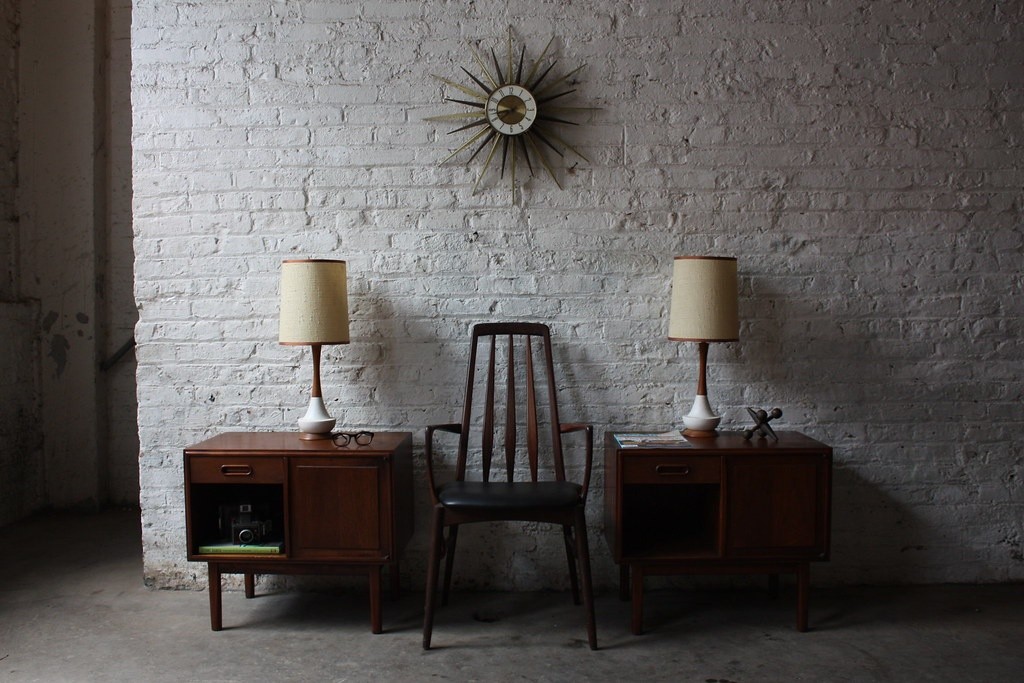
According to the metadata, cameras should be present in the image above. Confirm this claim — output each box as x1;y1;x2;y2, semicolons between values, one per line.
234;517;266;544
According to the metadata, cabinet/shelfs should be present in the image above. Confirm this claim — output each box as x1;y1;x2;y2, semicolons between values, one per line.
184;432;412;634
602;430;833;636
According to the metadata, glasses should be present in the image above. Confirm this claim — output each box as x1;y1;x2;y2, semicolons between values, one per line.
332;431;374;447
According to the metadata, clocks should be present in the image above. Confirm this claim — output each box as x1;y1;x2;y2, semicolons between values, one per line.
485;83;538;137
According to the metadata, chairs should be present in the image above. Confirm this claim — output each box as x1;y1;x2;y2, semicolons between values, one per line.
422;323;598;651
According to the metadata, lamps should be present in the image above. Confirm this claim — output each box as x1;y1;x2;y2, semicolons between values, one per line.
667;256;741;439
278;259;350;441
